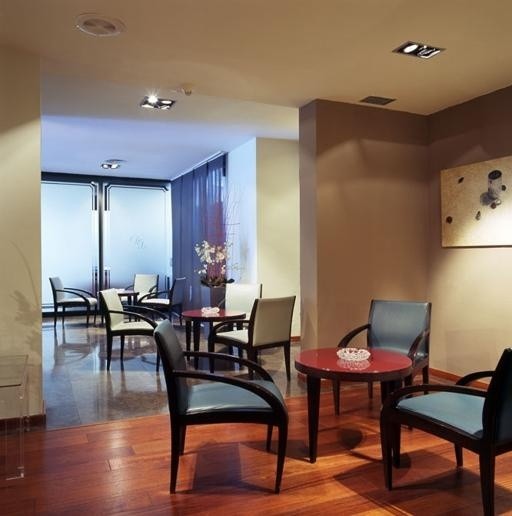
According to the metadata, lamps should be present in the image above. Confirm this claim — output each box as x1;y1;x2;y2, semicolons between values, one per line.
98;160;127;169
139;96;176;110
391;40;445;59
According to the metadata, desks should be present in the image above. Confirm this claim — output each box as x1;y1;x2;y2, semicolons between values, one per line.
0;354;31;482
294;347;414;469
182;309;247;375
96;290;139;326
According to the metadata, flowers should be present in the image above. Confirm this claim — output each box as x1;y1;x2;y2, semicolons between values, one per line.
191;239;240;289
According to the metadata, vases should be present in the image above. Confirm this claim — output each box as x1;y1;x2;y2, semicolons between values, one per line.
200;284;226;314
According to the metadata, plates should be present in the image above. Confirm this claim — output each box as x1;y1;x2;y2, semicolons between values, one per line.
337;348;371;361
201;313;220;317
337;359;370;371
201;307;220;313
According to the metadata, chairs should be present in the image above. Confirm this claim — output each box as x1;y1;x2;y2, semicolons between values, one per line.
138;277;186;326
209;283;263;354
125;273;159;322
49;276;98;328
378;347;512;516
207;295;296;382
99;288;170;374
153;317;290;495
334;299;432;430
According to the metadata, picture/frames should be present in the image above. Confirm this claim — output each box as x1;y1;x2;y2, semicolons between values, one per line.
440;155;512;248
